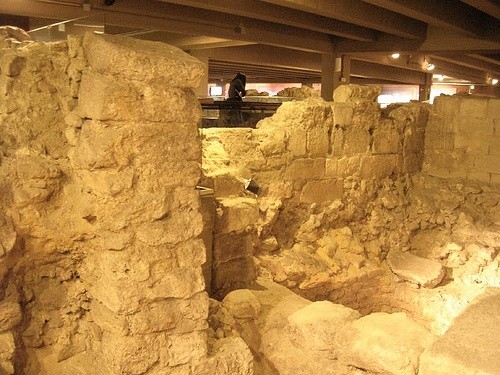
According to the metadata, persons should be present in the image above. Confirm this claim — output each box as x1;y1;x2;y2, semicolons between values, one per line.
229;73;246;109
